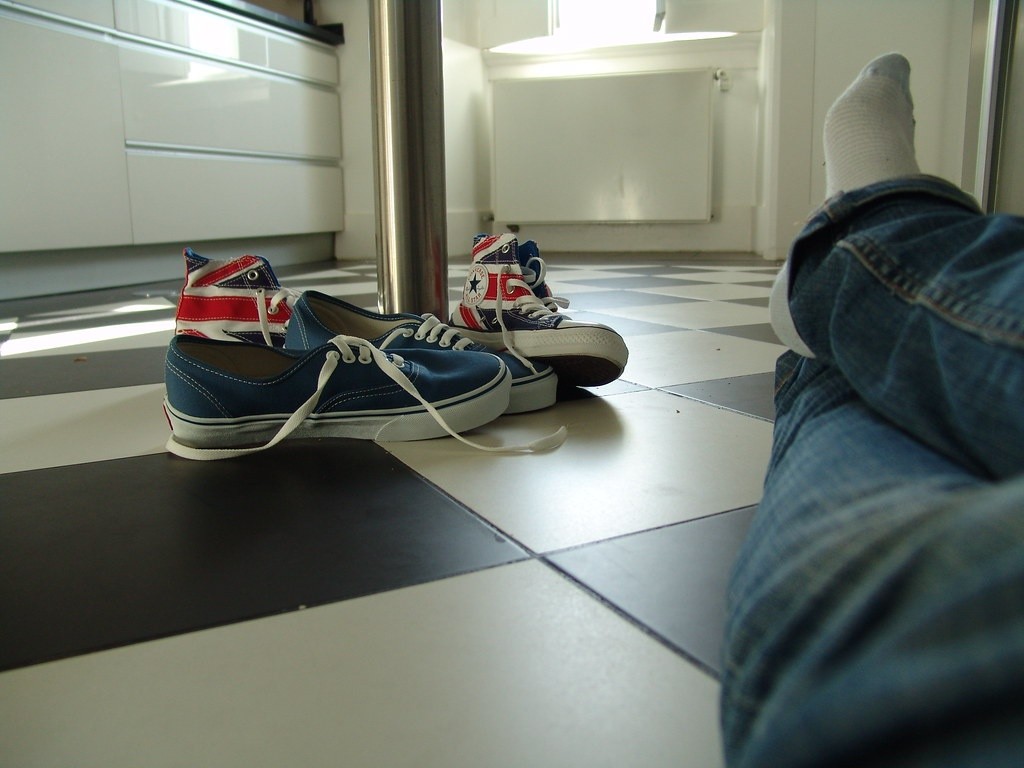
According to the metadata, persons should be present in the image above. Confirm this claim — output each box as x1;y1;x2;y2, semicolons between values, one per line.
721;53;1024;768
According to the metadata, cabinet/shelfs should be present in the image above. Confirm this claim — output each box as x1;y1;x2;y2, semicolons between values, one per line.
1;0;345;303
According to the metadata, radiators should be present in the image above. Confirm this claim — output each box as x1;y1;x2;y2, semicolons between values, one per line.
479;64;730;231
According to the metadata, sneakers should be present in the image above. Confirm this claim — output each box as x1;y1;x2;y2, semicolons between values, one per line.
447;233;629;387
282;290;558;414
175;248;302;350
162;334;512;460
